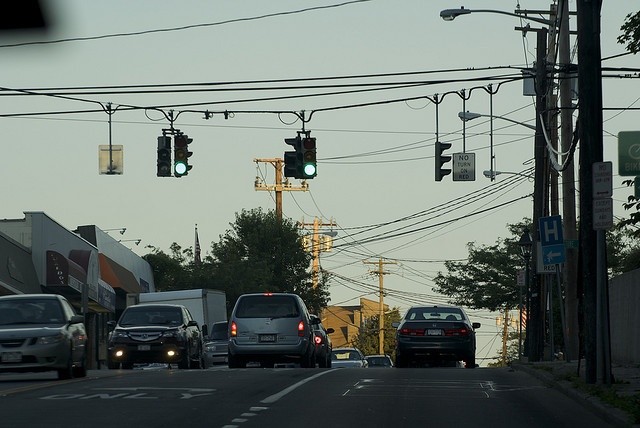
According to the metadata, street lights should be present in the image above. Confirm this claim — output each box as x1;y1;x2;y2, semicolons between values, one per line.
458;112;545;362
484;171;538;359
439;7;569;365
518;228;533;356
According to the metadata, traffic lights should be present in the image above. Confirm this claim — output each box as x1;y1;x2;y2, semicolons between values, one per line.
284;137;301;179
157;136;172;177
301;137;317;179
174;135;187;177
435;142;451;181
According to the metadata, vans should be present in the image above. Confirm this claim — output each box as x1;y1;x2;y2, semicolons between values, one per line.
228;293;321;370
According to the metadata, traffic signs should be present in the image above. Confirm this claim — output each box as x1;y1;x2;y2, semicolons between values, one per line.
539;215;566;264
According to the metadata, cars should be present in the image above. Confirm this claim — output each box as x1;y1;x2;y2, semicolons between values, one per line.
364;354;393;369
309;314;334;369
201;321;229;370
106;303;201;370
392;303;480;368
0;293;88;379
331;348;368;368
